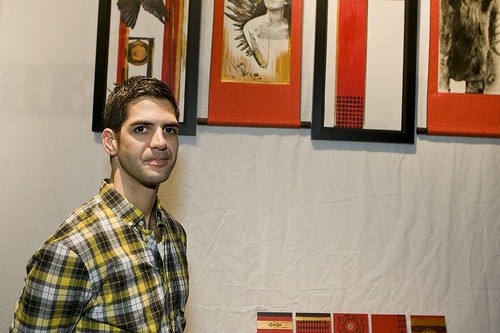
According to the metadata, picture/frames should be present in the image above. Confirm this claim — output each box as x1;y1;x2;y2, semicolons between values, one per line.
91;0;203;137
311;0;419;145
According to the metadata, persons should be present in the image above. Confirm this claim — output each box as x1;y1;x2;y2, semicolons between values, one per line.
10;75;189;333
